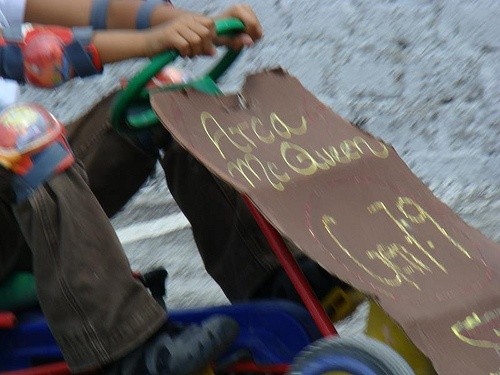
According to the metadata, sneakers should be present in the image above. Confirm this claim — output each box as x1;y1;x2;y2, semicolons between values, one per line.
251;254;331;307
99;313;240;375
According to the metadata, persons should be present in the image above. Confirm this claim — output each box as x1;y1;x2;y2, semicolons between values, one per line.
0;0;356;375
